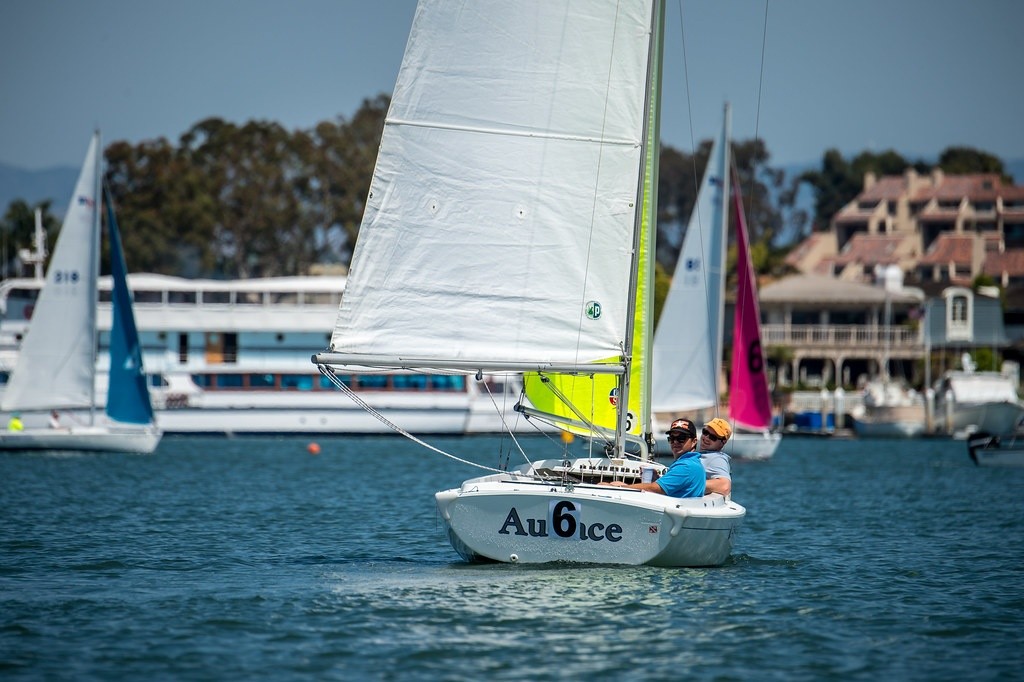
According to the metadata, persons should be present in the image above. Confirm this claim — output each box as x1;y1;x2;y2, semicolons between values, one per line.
48;410;60;428
611;418;732;494
7;413;24;431
596;418;705;497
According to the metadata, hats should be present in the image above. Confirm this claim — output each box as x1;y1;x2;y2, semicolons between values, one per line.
665;419;696;437
704;418;732;440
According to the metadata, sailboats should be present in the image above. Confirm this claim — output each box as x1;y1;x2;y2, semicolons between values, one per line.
310;0;745;567
594;101;785;462
0;129;164;454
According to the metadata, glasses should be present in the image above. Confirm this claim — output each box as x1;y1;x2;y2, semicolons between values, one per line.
702;428;725;441
667;435;695;443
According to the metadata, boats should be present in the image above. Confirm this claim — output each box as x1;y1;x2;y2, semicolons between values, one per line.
780;345;1024;466
0;206;554;434
